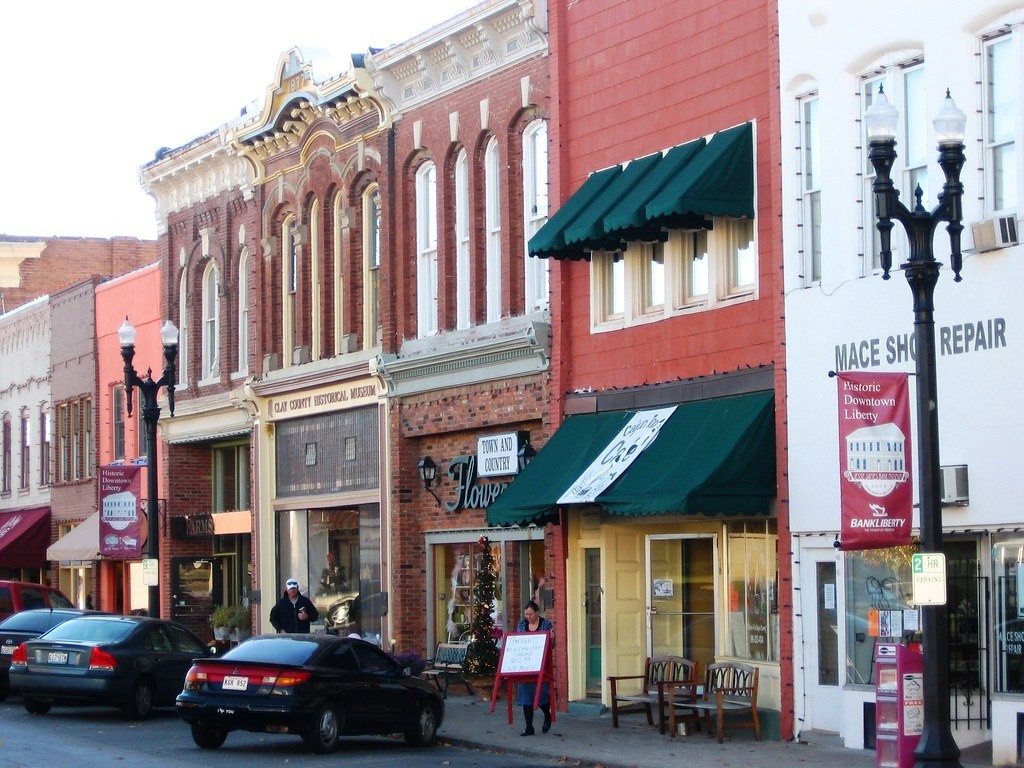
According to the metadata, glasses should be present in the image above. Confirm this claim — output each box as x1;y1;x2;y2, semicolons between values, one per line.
287;582;297;586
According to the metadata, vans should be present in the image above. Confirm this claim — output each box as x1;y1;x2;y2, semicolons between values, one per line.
0;580;77;621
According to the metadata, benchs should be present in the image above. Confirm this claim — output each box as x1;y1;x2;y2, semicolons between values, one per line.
422;642;470;698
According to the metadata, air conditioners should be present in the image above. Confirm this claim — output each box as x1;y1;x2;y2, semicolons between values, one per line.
970;215;1018;253
939;466;969;501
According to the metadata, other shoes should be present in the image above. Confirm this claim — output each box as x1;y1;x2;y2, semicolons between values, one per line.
542;714;551;733
520;727;534;736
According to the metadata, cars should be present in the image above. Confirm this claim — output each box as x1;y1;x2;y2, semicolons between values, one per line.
1;607;116;699
8;615;218;721
175;634;446;754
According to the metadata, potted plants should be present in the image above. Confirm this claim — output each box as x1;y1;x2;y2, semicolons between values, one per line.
207;606;250;641
459;535;503;703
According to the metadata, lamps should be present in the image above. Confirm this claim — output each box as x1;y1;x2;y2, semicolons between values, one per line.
519;440;537;471
417;454;441;507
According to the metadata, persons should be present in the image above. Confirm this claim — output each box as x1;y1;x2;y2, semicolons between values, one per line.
270;578;318;634
516;601;555;737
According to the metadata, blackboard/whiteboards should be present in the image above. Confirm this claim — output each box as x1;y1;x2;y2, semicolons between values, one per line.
497;631;551;676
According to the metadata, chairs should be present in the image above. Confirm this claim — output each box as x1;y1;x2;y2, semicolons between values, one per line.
607;654;702;735
667;660;763;744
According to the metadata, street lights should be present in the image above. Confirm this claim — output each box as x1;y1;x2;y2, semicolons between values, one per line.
118;314;180;620
864;81;967;768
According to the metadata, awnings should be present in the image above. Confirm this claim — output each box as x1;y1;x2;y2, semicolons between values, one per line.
604;138;706;242
564;152;662;251
528;165;623;261
646;121;754;230
485;390;777;526
45;507;149;561
0;507;52;568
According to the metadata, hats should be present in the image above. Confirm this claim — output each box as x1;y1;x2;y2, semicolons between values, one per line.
286;579;298;590
